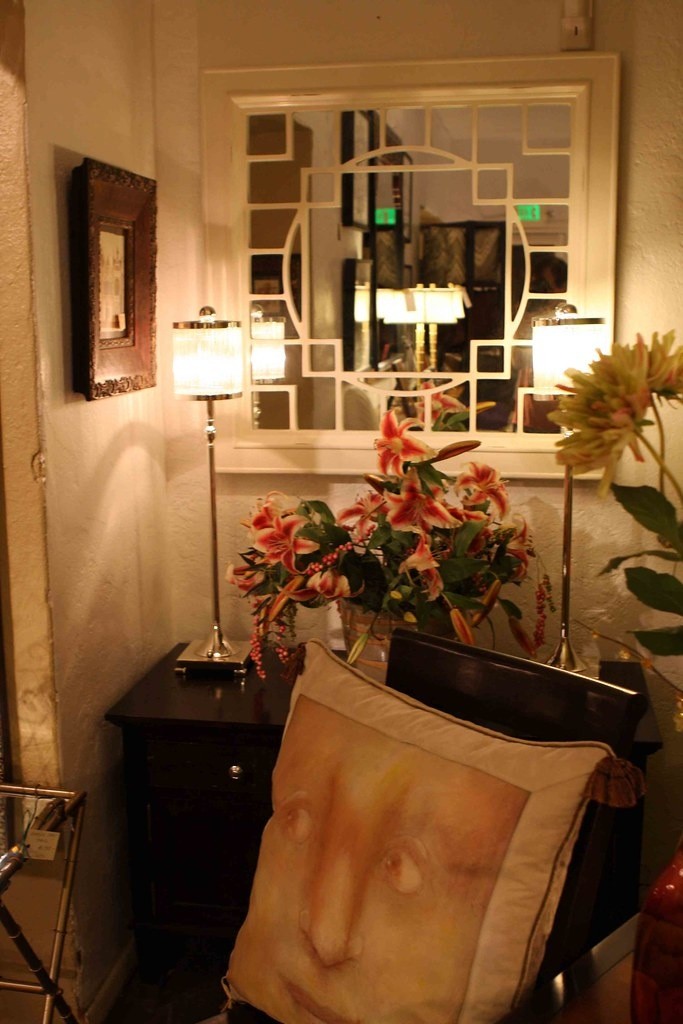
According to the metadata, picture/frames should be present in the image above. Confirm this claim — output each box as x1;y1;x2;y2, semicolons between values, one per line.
341;109;371;233
346;258;377;374
71;155;160;402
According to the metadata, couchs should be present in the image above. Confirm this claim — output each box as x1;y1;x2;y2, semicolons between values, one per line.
216;637;619;1024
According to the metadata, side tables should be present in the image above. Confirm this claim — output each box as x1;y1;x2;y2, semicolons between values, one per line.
100;640;358;946
581;657;663;913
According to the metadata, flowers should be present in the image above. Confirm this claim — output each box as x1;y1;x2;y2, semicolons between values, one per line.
547;328;683;727
224;409;549;680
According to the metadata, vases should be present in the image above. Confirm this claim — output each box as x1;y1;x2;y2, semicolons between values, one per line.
630;839;683;1024
332;597;447;683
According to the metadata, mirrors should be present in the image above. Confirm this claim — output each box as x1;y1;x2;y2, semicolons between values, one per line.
195;48;617;484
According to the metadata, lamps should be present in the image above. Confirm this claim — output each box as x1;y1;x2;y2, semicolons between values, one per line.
252;310;286;427
383;288;426;392
531;305;613;673
170;306;257;683
424;284;469;375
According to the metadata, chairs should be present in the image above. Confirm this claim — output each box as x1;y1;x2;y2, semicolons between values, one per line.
384;627;652;1024
